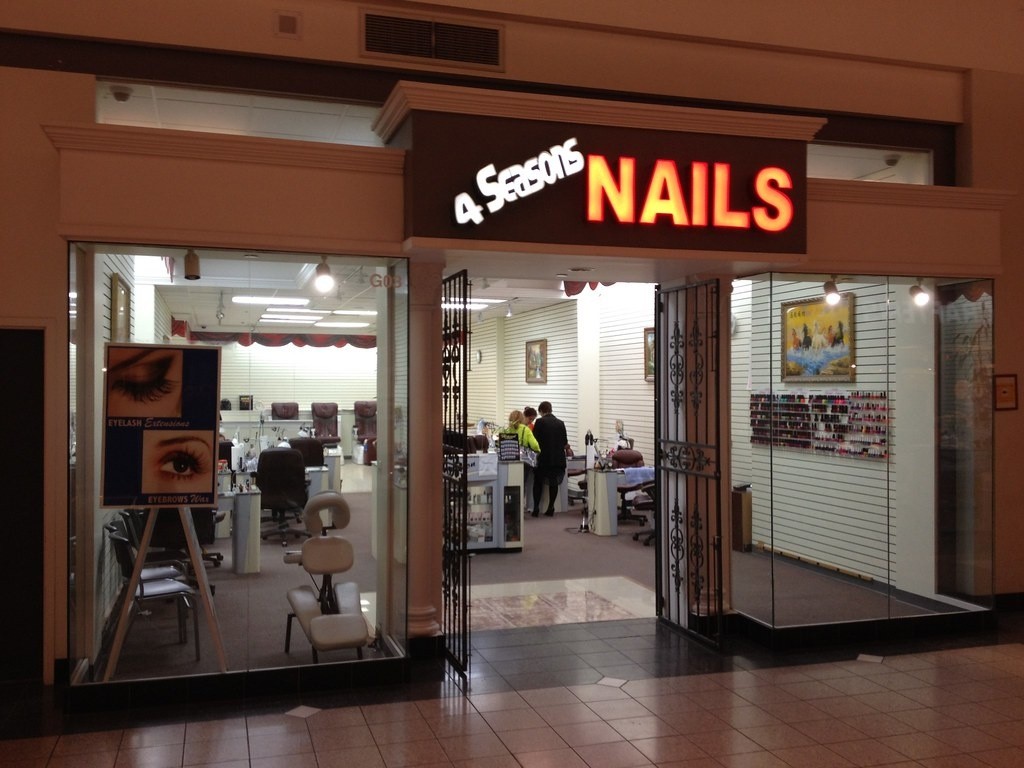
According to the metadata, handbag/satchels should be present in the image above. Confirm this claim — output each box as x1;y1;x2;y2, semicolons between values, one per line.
519;427;538;467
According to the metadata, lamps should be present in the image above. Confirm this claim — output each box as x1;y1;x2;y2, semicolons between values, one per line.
823;276;841;304
314;256;334;292
184;250;200;279
505;305;512;317
908;277;930;305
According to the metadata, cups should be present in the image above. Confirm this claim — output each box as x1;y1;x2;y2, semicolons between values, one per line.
467;493;514;542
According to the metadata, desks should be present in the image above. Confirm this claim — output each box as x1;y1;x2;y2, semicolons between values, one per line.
290;437;341;466
612;466;654;488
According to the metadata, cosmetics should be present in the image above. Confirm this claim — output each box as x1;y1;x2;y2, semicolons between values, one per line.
751;391;888;458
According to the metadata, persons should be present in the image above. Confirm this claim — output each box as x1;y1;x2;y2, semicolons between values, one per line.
530;402;569;518
142;431;213;493
108;349;182;417
504;411;541;466
523;407;537;432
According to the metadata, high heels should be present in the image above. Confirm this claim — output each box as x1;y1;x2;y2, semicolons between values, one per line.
544;508;555;517
531;507;540;517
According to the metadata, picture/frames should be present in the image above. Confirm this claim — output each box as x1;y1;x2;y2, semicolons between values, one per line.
110;273;131;342
780;292;856;382
525;338;546;383
643;327;655;381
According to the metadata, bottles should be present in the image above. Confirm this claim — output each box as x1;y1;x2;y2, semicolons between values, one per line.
218;460;229;474
477;418;495;449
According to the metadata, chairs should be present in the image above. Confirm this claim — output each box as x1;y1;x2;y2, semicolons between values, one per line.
255;448;312;546
567;450;656;545
313;402;346;467
271;402;299;421
351;398;380;465
106;510;201;665
